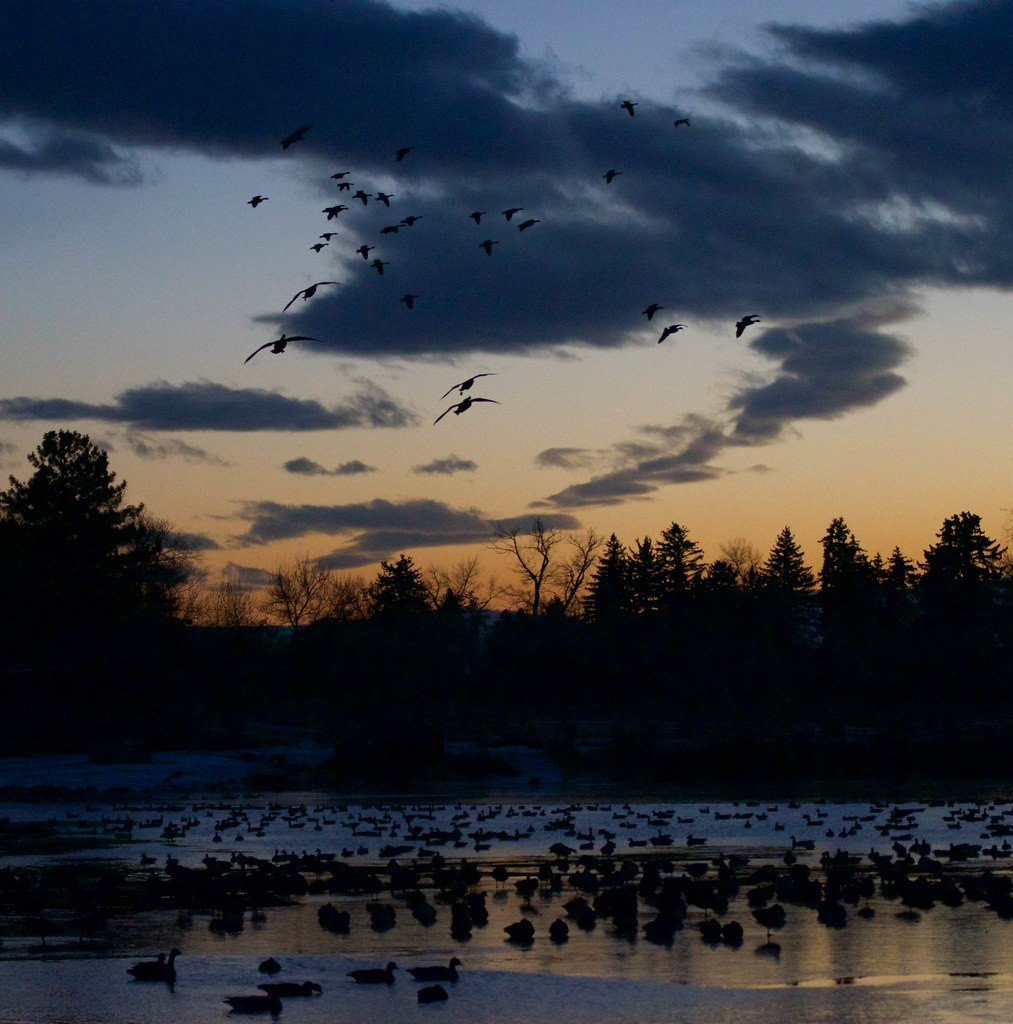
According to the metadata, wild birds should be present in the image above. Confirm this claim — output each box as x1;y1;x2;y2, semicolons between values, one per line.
319;233;338;242
322;205;349;221
247;195;269;208
603;169;622;184
7;796;1013;1018
338;182;394;207
282;281;341;312
244;334;323;363
432;396;502;425
735;314;761;338
396;147;415;163
658;325;688;344
356;208;541;276
309;243;329;253
674;118;692;127
331;171;351;179
642;304;664;320
620;101;639;116
441;373;496;399
400;294;420;310
280;126;310;151
